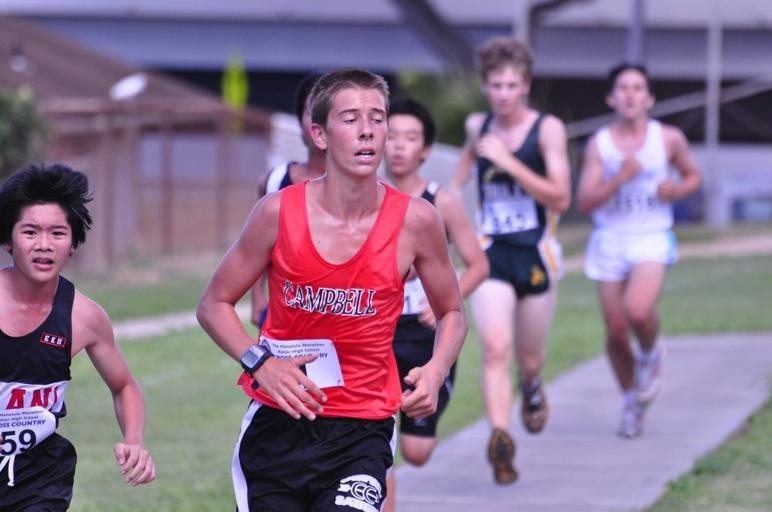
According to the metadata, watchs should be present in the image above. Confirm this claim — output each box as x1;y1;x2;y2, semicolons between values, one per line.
237;343;277;377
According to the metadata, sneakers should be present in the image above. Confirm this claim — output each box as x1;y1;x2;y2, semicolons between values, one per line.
518;376;546;433
616;404;646;439
489;429;517;485
633;339;665;405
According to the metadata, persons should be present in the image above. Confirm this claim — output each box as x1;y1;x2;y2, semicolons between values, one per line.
371;98;495;511
447;35;575;487
242;69;331;328
1;161;157;511
574;60;705;443
195;66;454;512
